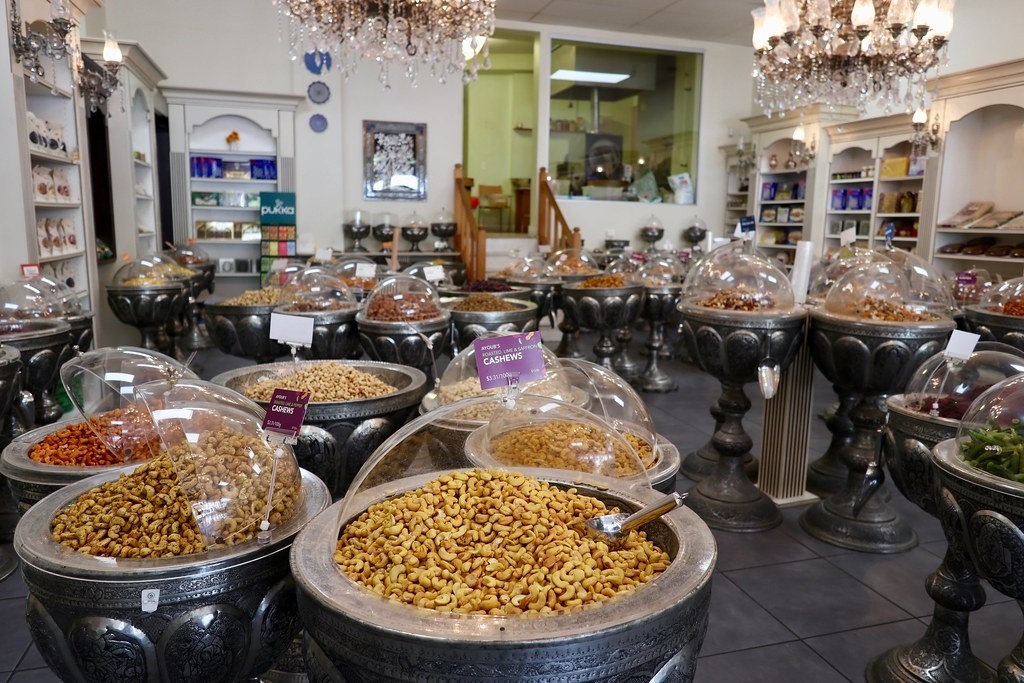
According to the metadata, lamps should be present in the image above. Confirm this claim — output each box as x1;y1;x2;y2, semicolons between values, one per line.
74;31;122;115
273;1;497;89
6;0;72;77
750;0;953;113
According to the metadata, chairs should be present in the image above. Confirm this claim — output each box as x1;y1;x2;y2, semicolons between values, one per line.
476;184;512;232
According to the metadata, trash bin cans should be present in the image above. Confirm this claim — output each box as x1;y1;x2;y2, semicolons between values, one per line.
514;189;530;233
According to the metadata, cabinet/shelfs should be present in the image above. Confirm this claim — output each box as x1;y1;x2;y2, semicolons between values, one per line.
0;0;163;347
715;56;1023;268
189;148;278;276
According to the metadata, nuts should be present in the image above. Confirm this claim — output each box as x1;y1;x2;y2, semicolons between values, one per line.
243;361;399;403
437;375;656;477
30;403;301;557
333;464;670;619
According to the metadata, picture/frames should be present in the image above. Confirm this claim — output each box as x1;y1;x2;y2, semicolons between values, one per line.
361;119;426;199
